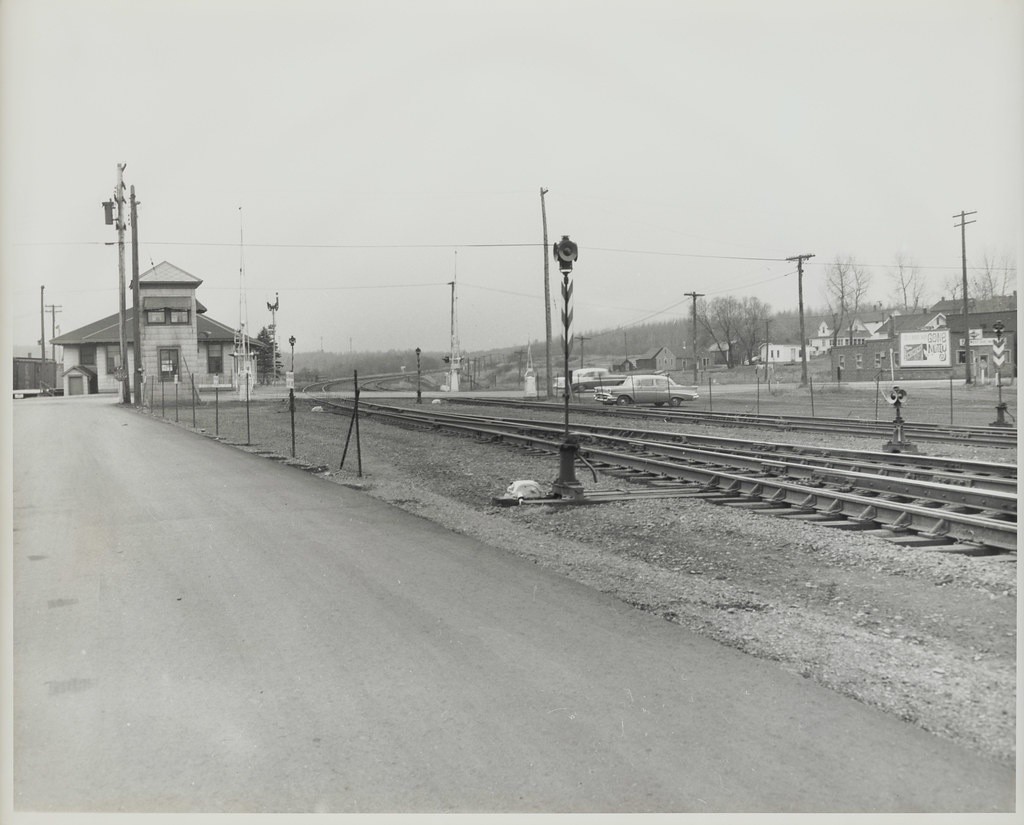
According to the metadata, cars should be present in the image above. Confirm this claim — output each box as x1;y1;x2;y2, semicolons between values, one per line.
593;375;699;407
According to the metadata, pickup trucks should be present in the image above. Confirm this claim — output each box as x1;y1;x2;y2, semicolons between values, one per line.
572;366;629;393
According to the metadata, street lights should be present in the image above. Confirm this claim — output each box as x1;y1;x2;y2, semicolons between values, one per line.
289;336;296;372
415;347;422;403
267;302;279;380
